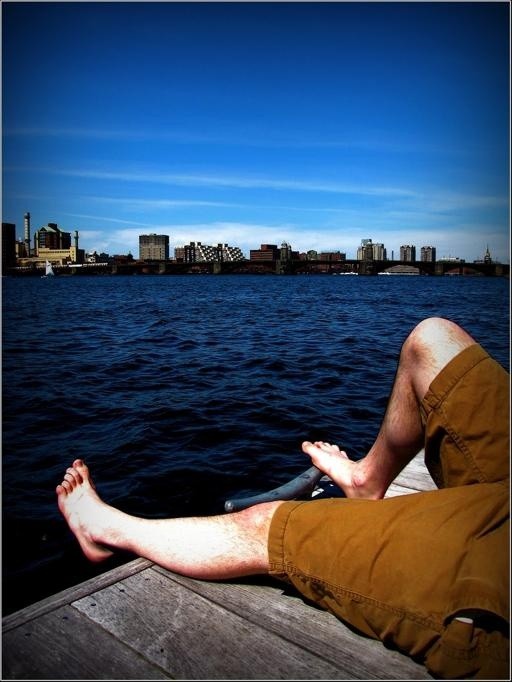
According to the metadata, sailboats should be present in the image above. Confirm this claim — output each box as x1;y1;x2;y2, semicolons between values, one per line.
40;258;55;280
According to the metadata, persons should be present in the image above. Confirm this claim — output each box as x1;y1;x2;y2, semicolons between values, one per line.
55;318;510;680
